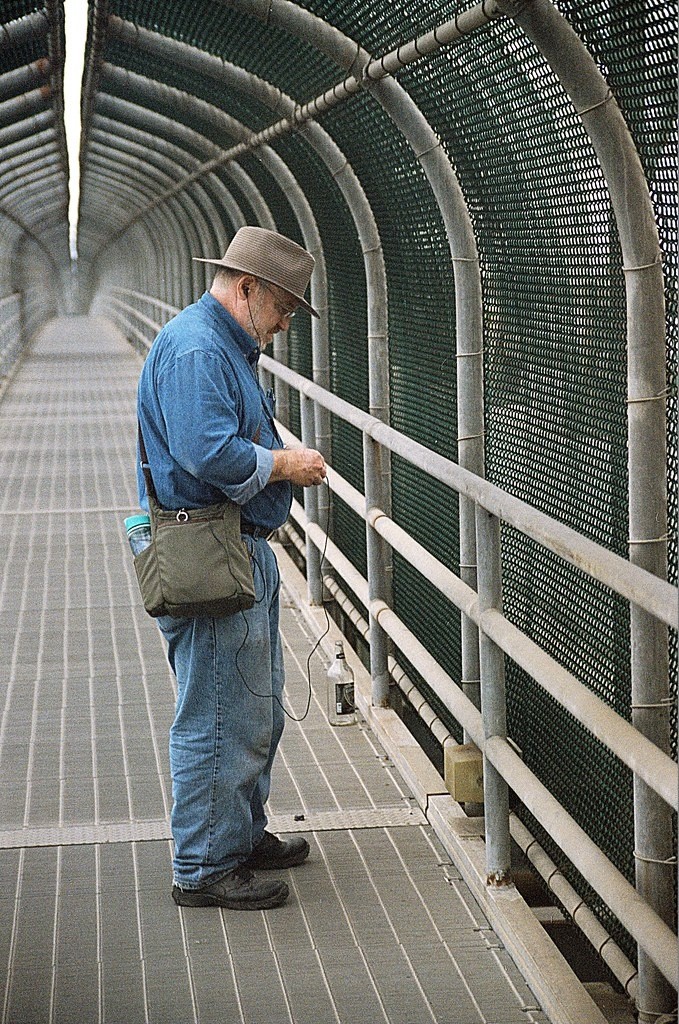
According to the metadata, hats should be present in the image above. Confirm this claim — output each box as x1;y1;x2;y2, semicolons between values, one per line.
189;222;324;325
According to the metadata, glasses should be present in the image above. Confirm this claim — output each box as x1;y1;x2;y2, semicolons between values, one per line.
261;280;296;319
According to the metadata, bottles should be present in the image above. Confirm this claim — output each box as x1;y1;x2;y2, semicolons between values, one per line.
326;640;356;726
124;515;153;557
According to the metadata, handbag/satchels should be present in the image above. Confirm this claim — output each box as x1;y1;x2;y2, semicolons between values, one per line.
130;495;253;618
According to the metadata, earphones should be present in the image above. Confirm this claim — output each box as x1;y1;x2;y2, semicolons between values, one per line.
242;286;249;297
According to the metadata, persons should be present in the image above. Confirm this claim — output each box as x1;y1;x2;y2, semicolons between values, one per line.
135;226;327;911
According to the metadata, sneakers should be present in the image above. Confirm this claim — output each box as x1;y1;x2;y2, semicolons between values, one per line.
171;863;290;911
242;830;311;870
